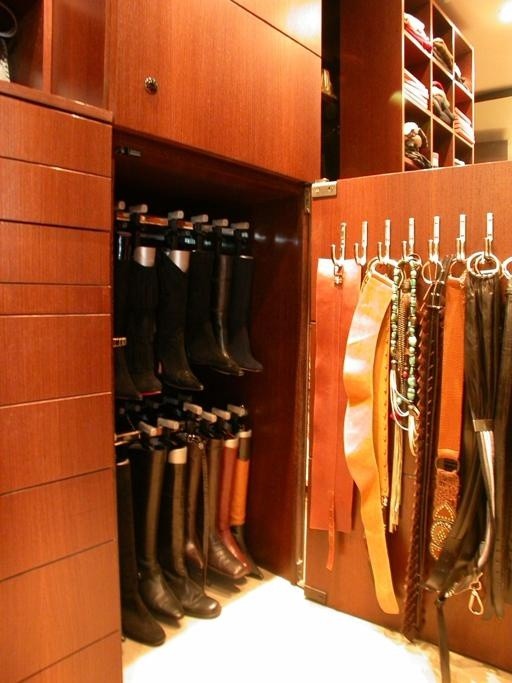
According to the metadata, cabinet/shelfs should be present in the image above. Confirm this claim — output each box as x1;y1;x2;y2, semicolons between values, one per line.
336;0;477;176
30;0;327;190
3;85;115;682
110;128;511;683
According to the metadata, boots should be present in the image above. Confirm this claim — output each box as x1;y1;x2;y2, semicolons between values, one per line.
112;247;264;401
115;417;263;646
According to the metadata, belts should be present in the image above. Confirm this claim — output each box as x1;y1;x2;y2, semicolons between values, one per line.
308;252;511;683
